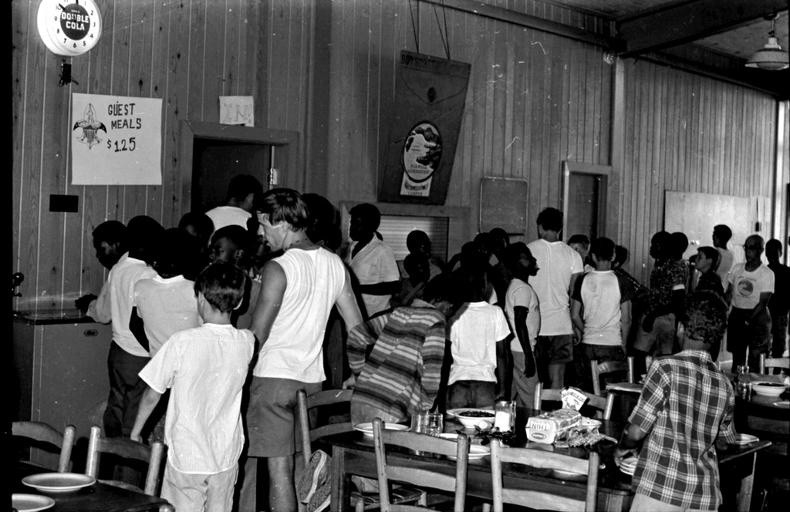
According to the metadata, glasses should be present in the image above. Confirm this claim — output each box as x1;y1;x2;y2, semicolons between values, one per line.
743;245;759;251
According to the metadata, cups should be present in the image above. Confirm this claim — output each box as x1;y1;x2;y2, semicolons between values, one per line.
410;413;443;457
496;400;517;434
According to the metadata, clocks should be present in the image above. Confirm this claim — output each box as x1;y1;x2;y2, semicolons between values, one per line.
37;0;103;57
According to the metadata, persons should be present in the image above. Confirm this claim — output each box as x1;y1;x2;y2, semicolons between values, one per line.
71;173;789;511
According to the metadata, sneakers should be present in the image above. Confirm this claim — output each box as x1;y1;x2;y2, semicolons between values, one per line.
307;477;331;511
298;449;331;504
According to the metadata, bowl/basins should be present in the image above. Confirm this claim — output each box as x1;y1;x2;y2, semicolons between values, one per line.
752;380;787;397
446;408;495;428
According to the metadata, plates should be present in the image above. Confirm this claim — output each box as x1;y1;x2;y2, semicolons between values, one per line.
553;462;606;477
447;444;491;459
735;433;759;445
352;422;409;436
773;401;790;408
21;472;96;493
11;492;55;512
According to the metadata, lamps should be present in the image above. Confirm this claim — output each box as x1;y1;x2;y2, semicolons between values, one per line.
745;13;790;71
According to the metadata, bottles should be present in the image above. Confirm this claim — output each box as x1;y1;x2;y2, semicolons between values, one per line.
737;366;751;401
